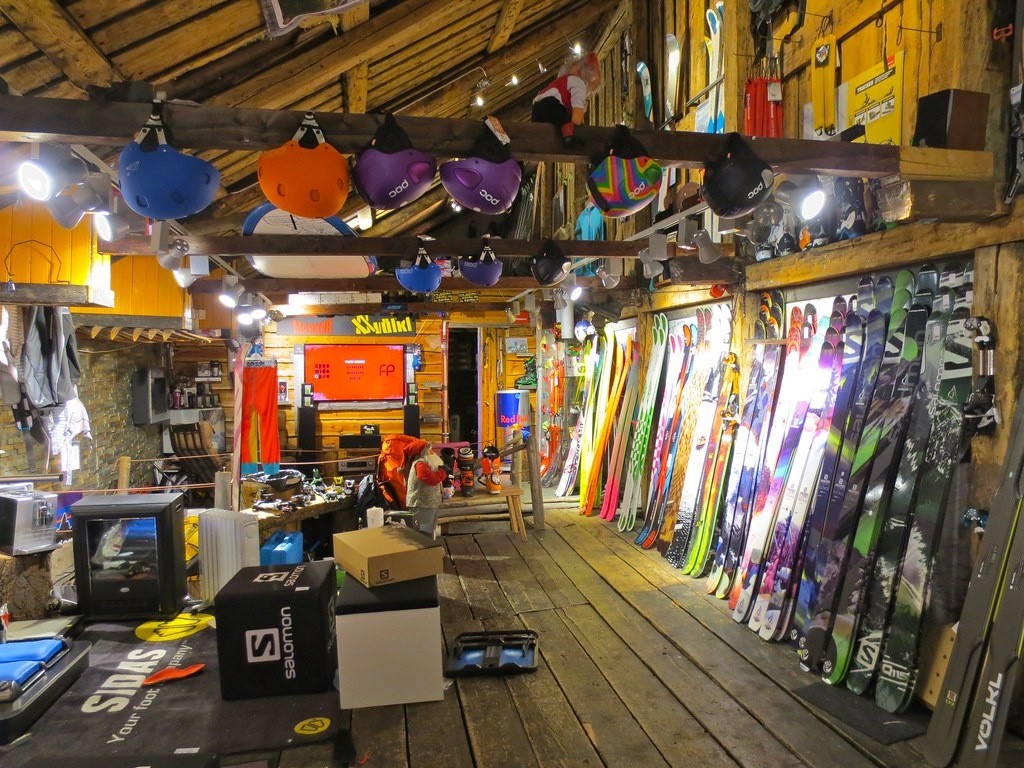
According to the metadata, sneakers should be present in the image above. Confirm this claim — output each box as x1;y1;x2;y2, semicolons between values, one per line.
756;246;774;261
801;217;828;252
774;231;797;258
832;177;866;241
869;174;902;232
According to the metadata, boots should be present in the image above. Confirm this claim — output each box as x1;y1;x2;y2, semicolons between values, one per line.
514;357;537;385
439;448;455;498
483;446;501;494
456;446;475;497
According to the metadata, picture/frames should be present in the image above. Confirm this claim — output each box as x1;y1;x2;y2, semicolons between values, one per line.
506;338;528;354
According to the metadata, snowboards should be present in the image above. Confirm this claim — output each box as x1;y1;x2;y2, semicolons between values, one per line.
240;201;378;280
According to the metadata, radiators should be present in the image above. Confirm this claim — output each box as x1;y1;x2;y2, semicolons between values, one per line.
198;508;260;605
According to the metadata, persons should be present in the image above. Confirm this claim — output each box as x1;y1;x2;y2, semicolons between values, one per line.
531;52;603;150
398;439;452;537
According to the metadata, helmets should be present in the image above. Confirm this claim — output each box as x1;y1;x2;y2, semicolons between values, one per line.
585;155;663;219
700;156;775;219
258;140;350;219
353;148;437;210
395;262;442;293
439;156;522;215
575;320;595;343
118;142;221;220
531;258;572;286
458;259;502;287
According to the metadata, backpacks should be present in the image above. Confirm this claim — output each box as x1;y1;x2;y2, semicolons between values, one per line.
376;434;418;510
356;475;400;517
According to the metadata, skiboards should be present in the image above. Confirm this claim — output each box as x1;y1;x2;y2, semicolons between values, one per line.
554;257;1024;768
630;2;722;246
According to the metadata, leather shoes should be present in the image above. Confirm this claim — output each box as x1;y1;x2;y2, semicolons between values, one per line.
588;300;624;323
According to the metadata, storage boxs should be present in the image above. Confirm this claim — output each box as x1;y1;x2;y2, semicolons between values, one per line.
333;525;444;588
214;559;338;702
335;572;443;710
260;530;303;566
69;494;187;621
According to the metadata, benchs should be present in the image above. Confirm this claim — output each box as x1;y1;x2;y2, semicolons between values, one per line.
431;484;529;542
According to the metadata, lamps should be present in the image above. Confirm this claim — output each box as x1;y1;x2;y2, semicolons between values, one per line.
563;279;582;301
173;268;210;288
596;268;621;289
157;239;190;270
45;172;111;230
639;249;664;279
92;212;147;242
775;180;827;221
692;228;722;264
219;283;246;308
18;157;88;202
753;193;783;226
237;293;283;327
553;293;568;310
735;223;771;244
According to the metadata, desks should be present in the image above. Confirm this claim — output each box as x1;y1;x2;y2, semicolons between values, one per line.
241;491;360;560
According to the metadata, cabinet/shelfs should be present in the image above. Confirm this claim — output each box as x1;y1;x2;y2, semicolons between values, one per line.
162;407;224;455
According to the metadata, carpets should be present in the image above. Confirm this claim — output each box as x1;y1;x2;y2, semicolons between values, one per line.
0;620;338;768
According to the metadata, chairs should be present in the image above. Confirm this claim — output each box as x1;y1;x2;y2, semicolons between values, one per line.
167;421;262;508
276;410;300;471
